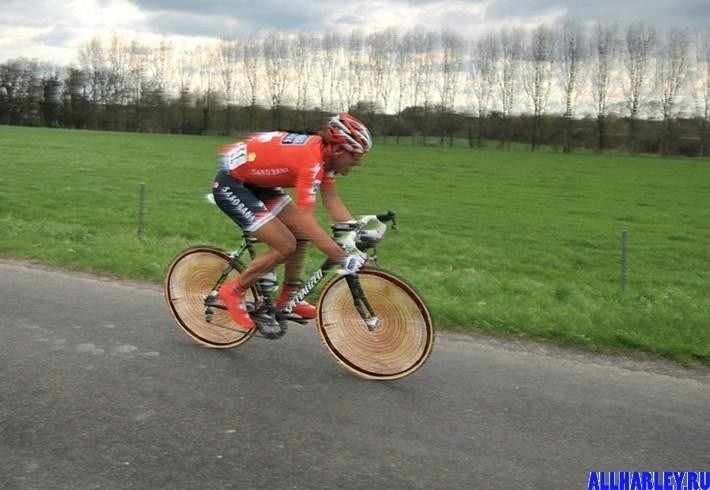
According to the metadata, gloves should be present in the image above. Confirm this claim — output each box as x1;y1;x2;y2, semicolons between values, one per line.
345;255;365;276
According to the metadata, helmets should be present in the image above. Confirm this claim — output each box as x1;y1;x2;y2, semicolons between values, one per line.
327;113;373;154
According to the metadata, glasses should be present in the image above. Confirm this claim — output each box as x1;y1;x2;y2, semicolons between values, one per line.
346;149;363;162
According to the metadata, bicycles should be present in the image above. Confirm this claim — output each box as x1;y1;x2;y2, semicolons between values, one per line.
164;194;435;382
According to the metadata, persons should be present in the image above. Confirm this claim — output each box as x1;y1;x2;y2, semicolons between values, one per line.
212;112;372;329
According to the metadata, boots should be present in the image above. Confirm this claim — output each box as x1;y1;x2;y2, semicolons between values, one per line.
278;285;317;318
219;278;256;328
